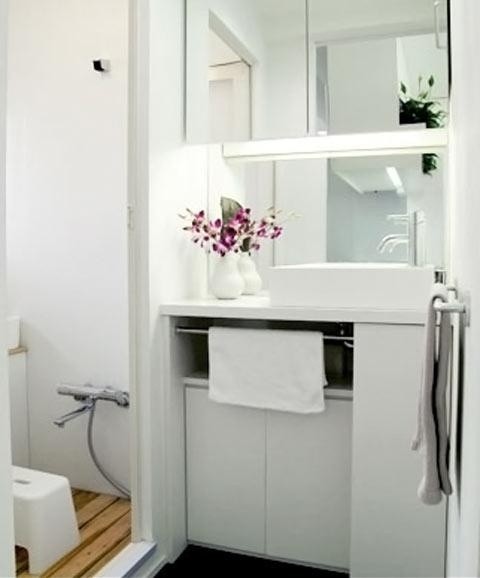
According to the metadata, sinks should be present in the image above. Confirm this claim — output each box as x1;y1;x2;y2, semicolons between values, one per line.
272;263;435;309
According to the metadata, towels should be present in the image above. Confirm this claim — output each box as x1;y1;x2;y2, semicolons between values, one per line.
206;326;329;415
409;281;454;508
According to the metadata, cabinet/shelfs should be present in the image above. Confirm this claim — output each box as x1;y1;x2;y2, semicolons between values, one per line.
184;387;352;569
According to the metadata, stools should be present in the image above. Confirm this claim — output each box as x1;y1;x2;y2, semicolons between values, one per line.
11;462;81;575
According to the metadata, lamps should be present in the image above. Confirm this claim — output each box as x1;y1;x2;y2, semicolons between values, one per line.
219;0;447;164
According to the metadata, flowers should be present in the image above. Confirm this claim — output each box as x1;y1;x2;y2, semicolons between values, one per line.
178;196;274;255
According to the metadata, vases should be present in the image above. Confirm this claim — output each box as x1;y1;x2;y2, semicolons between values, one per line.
210;255;243;300
237;252;262;297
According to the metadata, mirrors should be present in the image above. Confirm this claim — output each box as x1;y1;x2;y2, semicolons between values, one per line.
186;0;451;306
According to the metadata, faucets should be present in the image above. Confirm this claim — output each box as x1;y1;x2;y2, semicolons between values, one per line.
50;384;131;429
374;211;420;266
